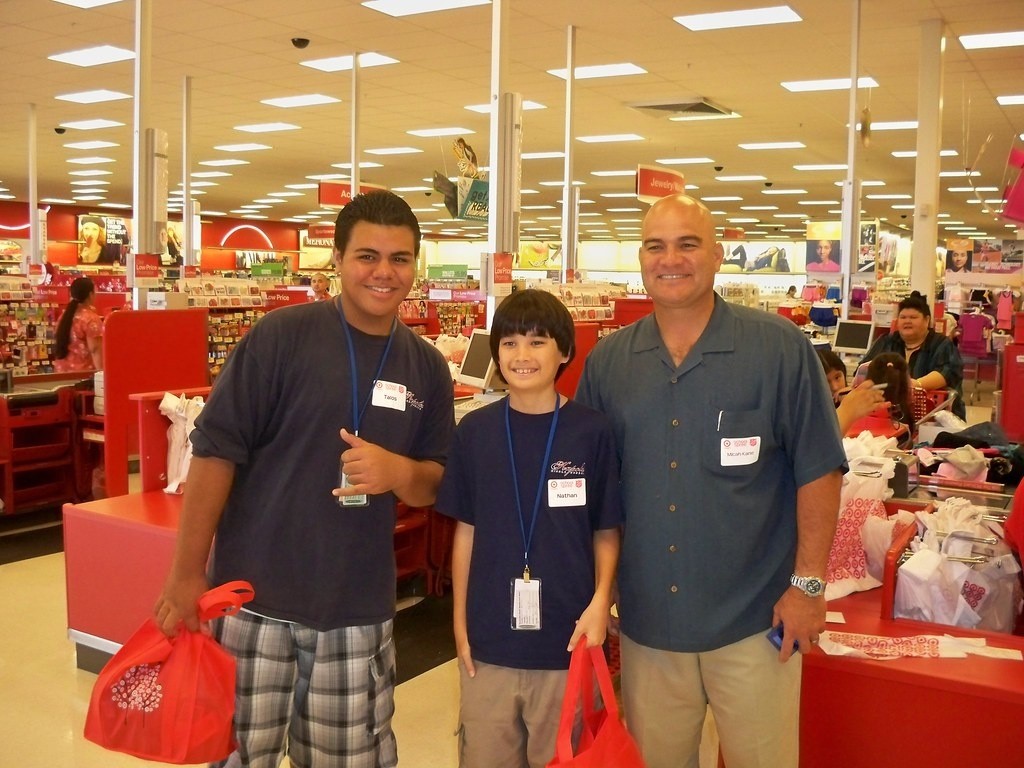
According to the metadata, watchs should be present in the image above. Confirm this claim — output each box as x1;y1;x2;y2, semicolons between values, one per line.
788;574;828;597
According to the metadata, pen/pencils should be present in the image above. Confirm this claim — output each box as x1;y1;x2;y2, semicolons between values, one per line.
717;410;725;432
839;382;889;396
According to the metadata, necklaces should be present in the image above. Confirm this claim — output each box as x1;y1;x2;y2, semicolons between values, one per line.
904;344;921;351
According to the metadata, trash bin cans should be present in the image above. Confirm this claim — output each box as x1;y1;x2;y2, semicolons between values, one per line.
991;390;1003;425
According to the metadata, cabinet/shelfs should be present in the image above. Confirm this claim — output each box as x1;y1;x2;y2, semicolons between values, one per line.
0;266;1024;768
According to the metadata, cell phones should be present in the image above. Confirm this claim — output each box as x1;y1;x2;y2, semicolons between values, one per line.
766;620;799;658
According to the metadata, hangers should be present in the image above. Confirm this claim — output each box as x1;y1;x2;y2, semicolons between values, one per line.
972;282;991;291
943;280;969;294
969;306;983;316
803;278;826;288
851;279;870;289
828;280;840;288
995;285;1020;297
993;330;1012;338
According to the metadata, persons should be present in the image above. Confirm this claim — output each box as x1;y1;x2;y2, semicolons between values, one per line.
572;194;849;768
308;272;332;302
854;290;965;422
54;278;104;372
813;349;915;447
154;187;456;768
719;245;779;271
166;226;183;265
80;216;111;263
946;250;971;273
806;239;840;272
785;285;796;300
431;288;623;768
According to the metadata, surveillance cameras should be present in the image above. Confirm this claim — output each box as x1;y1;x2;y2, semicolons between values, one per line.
900;215;907;219
714;166;723;171
291;38;309;49
54;128;65;135
425;193;432;196
765;183;773;187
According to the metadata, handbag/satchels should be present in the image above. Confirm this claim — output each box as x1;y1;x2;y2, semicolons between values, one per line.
543;638;647;768
83;580;254;765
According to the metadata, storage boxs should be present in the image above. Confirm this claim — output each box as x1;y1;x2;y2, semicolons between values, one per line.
870;303;897;329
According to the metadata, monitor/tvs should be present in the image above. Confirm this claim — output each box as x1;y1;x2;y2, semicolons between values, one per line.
457;328;496;389
831;320;876;354
